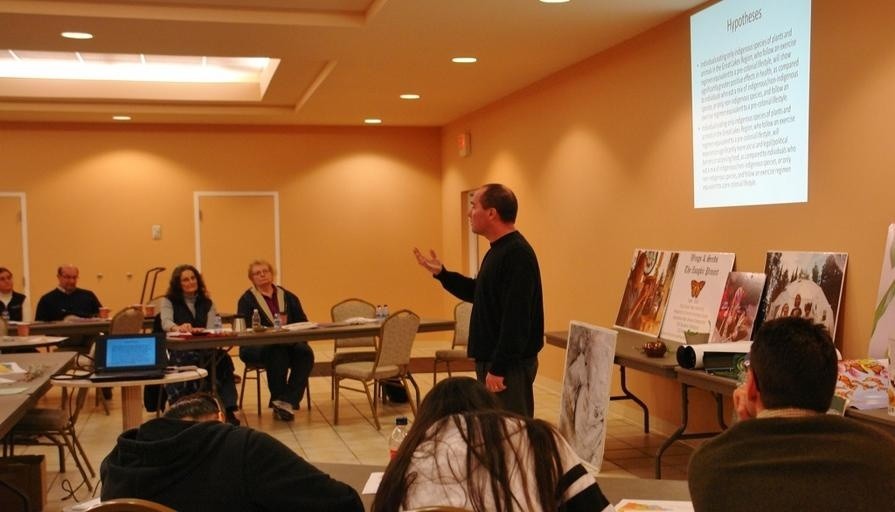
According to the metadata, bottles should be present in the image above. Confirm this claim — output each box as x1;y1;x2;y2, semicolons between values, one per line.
252;309;261;328
274;313;281;329
376;304;384;324
388;417;410;463
382;304;390;324
214;314;222;331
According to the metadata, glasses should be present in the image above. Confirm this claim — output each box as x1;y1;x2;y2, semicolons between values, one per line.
61;274;79;280
742;359;759;389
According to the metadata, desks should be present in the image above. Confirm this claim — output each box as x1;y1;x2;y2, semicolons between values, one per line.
1;313;238;352
0;353;80;473
161;315;453;393
50;366;208;433
308;462;695;512
545;327;895;481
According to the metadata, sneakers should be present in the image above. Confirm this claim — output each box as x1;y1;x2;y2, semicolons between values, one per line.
272;400;294;420
273;409;282;421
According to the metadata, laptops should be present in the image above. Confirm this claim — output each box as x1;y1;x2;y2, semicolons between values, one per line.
89;333;166;382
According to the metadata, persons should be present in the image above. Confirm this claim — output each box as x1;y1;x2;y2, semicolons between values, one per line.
237;260;314;421
0;268;40;354
687;317;895;512
790;294;803;318
781;303;790;318
615;253;653;333
412;184;543;419
160;264;240;427
803;303;813;319
99;393;365;512
371;376;615;512
34;265;113;400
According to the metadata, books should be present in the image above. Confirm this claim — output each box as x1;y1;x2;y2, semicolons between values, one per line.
343;317;381;324
75;497;101;512
165;332;192;340
282;322;318;331
6;321;31;325
204;328;267;333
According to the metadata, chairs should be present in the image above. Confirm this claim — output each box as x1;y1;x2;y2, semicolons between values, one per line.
239;363;312;417
334;309;421;430
93;305;144;408
86;499;172;512
51;331;110;416
330;299;381;400
0;351;95;492
433;301;474;385
404;506;468;512
